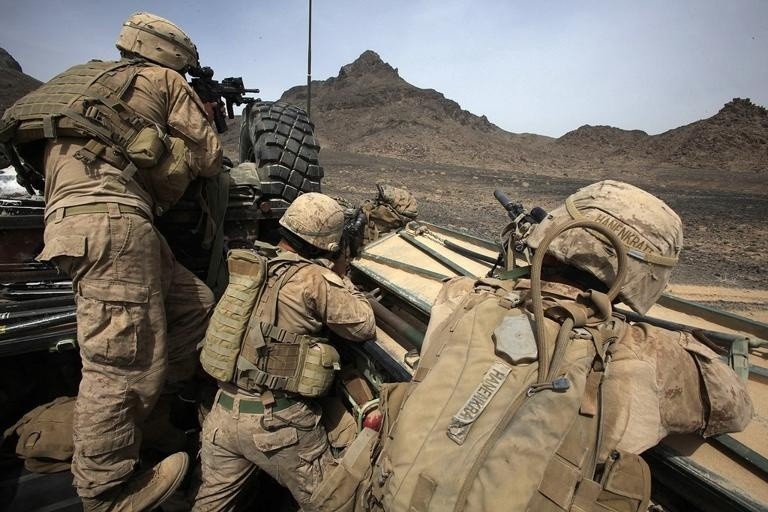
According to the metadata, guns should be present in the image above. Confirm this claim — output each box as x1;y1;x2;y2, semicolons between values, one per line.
187;66;260;132
486;190;547;278
340;211;368;257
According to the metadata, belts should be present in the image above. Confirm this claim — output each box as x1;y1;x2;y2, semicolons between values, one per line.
216;392;297;415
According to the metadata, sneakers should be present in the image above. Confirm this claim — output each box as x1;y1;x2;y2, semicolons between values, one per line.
84;452;188;510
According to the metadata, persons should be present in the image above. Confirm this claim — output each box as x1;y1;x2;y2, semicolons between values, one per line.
188;191;377;512
0;12;237;512
306;175;754;512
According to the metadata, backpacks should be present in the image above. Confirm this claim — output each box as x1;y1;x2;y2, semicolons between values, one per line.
371;278;596;511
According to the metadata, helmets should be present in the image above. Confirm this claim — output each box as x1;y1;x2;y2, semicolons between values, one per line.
278;191;343;256
113;11;198;75
526;178;685;318
